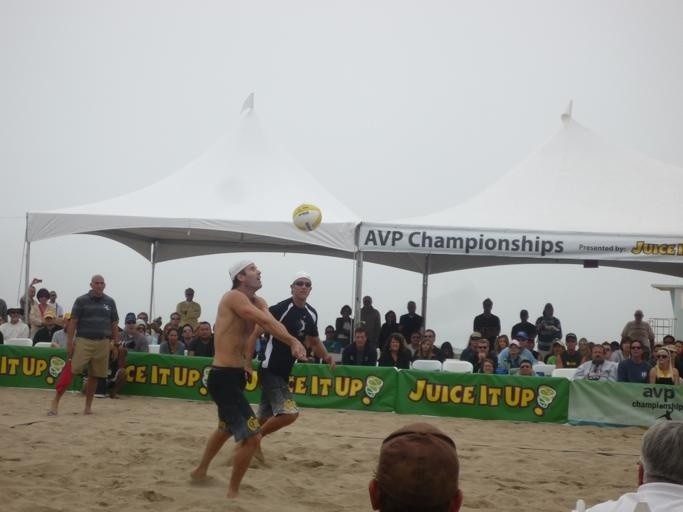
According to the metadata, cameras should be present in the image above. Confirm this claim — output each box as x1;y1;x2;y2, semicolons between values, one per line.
37;280;42;282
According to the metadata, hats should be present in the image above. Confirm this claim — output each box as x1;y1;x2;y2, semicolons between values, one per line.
288;272;312;284
227;257;253;281
325;297;417;344
42;311;55;319
63;311;71;320
470;298;577;351
37;288;51;300
125;311;162;333
7;306;24;316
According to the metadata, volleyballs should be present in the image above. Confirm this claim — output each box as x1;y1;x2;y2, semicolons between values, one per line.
293;204;321;229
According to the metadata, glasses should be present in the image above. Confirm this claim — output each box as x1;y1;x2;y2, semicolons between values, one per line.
520;362;530;371
293;281;311;287
629;345;668;360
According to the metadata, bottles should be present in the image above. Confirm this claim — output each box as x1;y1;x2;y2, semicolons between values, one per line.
183;346;188;356
151;336;157;345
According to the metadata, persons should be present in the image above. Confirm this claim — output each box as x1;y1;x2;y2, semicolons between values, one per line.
189;259;308;498
81;344;127;401
571;417;683;512
243;273;338;466
48;275;119;415
368;420;461;510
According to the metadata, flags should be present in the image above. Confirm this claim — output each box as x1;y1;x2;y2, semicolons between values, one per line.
53;359;71;391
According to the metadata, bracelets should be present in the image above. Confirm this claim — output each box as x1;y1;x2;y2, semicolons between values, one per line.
113;343;119;347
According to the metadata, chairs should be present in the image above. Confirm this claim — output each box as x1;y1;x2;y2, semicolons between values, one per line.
318;352;343;364
552;368;581;380
411;359;443;374
35;342;51;348
533;364;556;377
8;338;33;346
148;345;189;356
441;359;473;374
